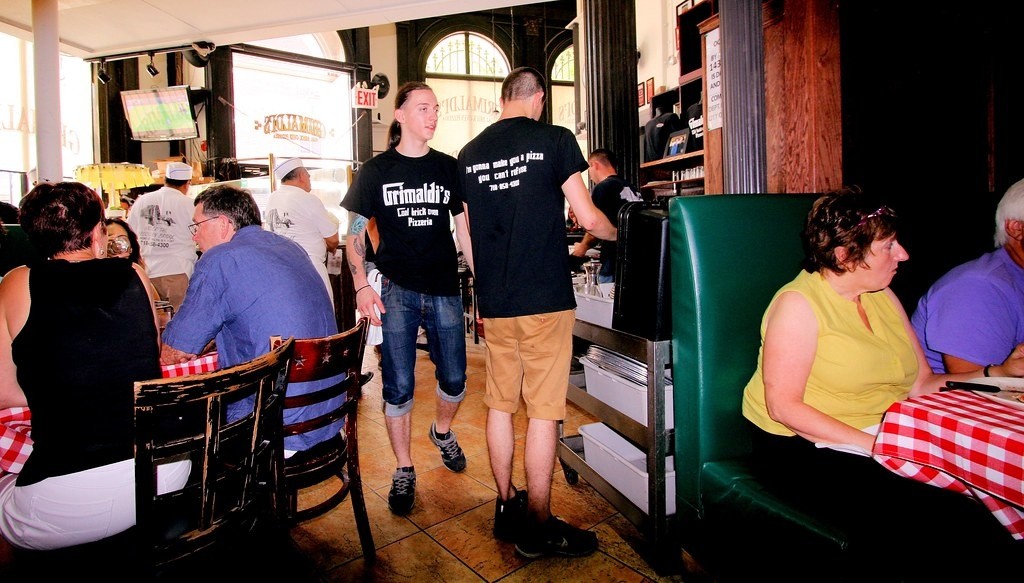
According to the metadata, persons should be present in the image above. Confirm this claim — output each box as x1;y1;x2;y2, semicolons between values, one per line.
0;158;381;389
566;149;647;285
161;185;346;532
457;67;618;559
910;178;1024;374
741;190;1024;583
0;183;161;583
340;82;475;518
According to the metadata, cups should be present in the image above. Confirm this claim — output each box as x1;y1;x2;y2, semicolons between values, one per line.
107;237;129;258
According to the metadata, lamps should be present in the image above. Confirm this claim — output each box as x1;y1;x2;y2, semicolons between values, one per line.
146;50;159;78
97;57;112;85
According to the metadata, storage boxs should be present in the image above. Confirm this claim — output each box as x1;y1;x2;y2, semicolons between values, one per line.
573;290;677;516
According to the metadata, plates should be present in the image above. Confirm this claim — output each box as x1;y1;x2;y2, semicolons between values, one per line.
968;377;1024;410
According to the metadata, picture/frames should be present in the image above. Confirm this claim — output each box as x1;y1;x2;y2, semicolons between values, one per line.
638;82;645;108
646;77;654;104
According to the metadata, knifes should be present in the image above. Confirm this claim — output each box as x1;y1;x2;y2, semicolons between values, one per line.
946;381;1024;394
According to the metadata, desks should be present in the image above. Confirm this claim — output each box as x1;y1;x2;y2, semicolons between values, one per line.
871;377;1024;583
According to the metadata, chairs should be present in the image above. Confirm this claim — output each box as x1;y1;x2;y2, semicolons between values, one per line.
9;244;380;583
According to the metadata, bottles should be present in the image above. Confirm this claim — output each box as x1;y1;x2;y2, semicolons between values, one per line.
579;262;603;298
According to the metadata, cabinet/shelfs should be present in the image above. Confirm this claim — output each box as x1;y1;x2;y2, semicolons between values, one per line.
557;313;682;579
695;11;724;196
640;149;704;202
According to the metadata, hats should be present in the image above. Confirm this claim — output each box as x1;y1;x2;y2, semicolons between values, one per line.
274;156;303;182
166;162;192;180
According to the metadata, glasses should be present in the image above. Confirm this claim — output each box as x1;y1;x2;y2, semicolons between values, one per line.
188;216;231;237
834;205;898;232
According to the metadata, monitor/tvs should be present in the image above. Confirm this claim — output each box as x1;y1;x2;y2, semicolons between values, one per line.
120;85;200;143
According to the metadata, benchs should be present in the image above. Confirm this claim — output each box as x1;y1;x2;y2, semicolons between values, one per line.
667;192;934;583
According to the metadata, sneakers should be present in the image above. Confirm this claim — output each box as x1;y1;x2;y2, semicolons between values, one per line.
388;467;417;516
493;485;528;543
514;516;599;560
429;422;468;473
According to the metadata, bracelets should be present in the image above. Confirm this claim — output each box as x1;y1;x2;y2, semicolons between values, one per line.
984;364;1001;377
356;285;371;295
